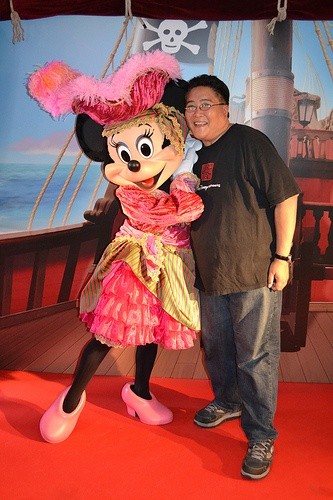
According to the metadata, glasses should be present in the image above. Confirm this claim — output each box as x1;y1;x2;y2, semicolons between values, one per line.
184;102;230;112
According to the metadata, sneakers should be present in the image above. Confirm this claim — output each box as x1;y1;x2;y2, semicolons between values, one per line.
193;399;242;427
240;439;274;479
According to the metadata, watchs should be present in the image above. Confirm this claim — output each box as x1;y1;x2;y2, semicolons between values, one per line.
275;252;293;263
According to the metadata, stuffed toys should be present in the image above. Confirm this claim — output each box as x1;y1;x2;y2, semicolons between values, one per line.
40;49;205;443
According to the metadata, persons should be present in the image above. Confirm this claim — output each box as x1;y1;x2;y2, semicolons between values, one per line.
184;74;305;477
